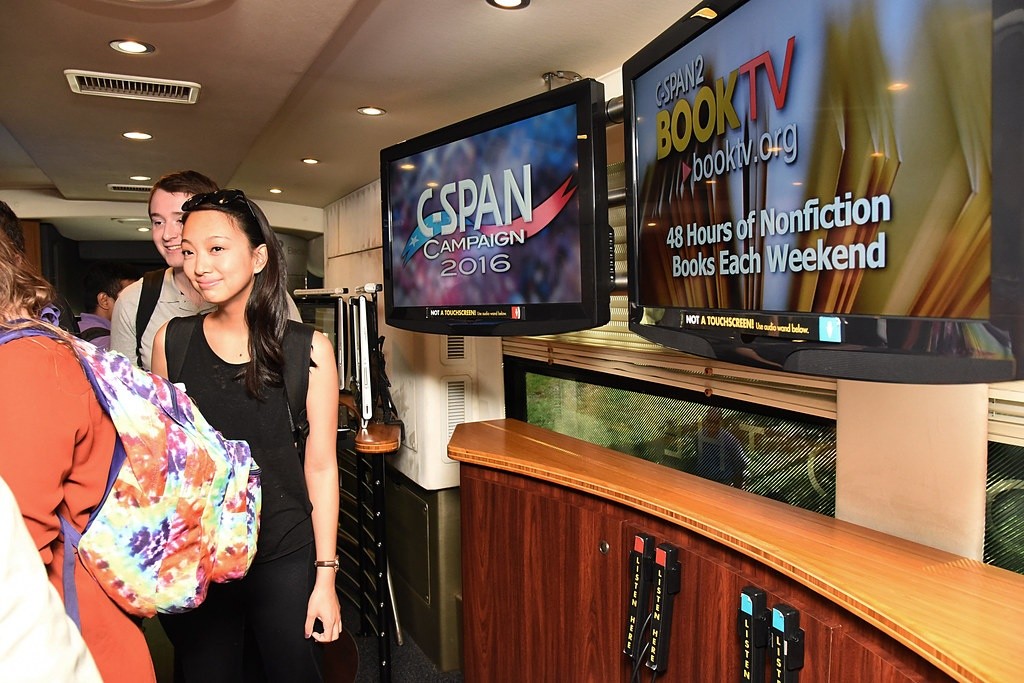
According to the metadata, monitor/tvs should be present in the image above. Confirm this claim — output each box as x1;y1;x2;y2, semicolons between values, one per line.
298;295;378;429
380;78;611;337
621;0;1024;384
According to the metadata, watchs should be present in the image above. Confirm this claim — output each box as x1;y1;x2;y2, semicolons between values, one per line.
315;555;340;572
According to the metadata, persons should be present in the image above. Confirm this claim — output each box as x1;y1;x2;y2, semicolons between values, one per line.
0;170;345;683
681;405;748;487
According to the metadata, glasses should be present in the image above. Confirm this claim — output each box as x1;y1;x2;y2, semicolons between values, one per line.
182;190;257;221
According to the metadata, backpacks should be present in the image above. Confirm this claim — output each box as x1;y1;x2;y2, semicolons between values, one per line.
0;320;264;618
